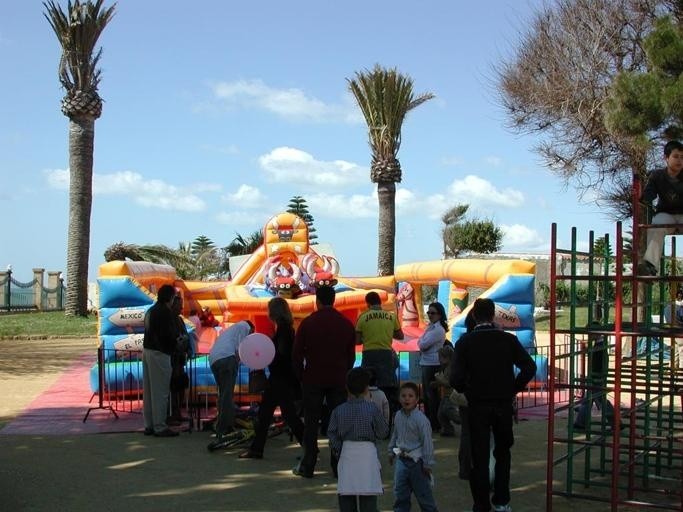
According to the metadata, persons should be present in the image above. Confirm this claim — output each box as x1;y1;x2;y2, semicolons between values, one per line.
291;285;356;478
635;140;683;285
326;366;390;512
435;345;462;438
456;308;477;481
366;367;389;425
207;319;255;442
662;288;683;380
573;322;619;434
387;382;439;512
166;296;194;427
354;292;404;395
449;306;475;481
417;302;449;434
448;298;538;512
141;283;179;438
238;297;320;461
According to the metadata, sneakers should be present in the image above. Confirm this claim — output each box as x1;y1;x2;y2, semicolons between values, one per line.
492;499;510;512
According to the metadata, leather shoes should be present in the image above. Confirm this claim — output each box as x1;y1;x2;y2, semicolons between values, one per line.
144;428;152;435
167;418;179;425
155;430;178;436
239;451;261;458
178;417;191;421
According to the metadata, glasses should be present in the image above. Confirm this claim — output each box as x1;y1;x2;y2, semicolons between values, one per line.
427;311;438;314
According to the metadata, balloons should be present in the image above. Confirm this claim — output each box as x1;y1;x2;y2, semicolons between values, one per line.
237;332;276;372
